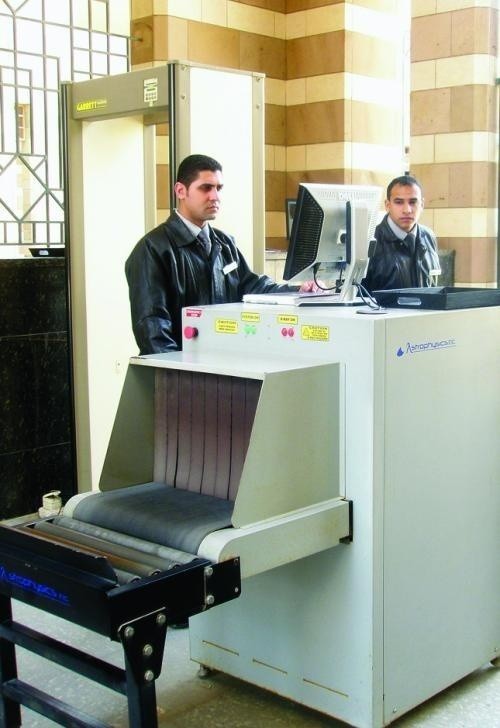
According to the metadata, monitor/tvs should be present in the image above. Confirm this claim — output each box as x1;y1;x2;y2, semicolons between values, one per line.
283;183;383;307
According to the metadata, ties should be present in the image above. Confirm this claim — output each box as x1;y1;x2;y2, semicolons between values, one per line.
199;230;212;256
407;233;415;256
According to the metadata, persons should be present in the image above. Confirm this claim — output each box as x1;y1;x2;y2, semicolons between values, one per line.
356;174;442;297
124;154;329;356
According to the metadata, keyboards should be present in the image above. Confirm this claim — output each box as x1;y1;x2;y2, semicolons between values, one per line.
242;292;341;305
